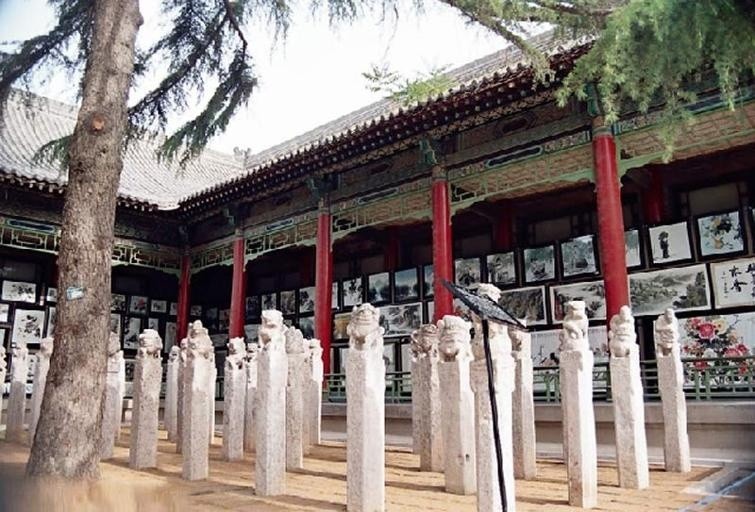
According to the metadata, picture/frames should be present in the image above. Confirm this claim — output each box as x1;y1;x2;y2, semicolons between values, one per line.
1;203;755;400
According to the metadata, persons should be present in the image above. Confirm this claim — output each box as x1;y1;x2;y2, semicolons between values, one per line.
529;256;549;279
569;244;588;269
